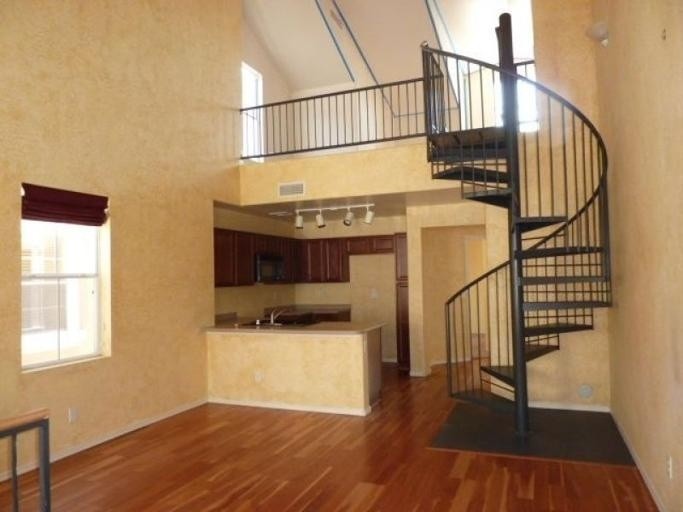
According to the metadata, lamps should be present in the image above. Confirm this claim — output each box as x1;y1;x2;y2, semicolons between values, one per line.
295;203;375;230
586;23;609;48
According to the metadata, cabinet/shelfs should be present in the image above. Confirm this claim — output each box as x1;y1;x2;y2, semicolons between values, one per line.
208;228;395;325
395;233;409;373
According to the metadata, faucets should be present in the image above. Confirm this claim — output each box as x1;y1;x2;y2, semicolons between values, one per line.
269;306;289;324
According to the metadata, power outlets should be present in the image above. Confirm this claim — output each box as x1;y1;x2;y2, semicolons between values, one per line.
665;454;672;480
68;406;79;422
